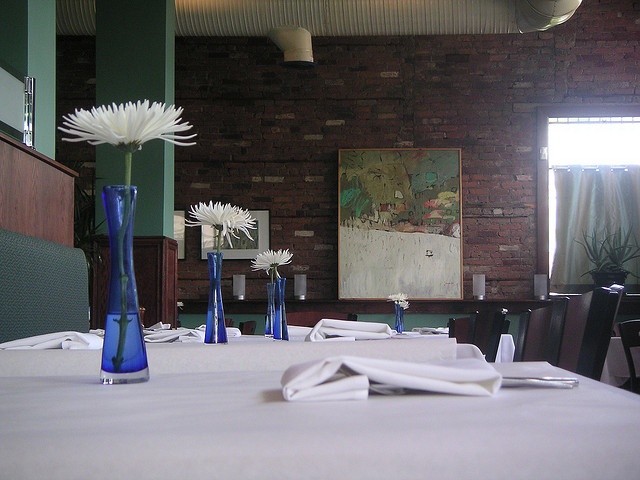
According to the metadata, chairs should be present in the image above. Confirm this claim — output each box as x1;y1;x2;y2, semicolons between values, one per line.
239;320;256;335
514;303;559;367
473;310;505;362
226;317;232;327
449;318;473;344
617;319;640;394
559;288;619;381
501;320;510;334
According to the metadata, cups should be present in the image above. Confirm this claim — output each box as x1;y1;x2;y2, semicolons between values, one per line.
473;274;485;302
533;274;548;301
233;275;246;300
294;275;306;300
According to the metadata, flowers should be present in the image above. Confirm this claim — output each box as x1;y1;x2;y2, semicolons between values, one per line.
184;200;257;340
57;98;199;369
387;292;408;304
250;248;294;303
250;261;274;304
399;300;410;310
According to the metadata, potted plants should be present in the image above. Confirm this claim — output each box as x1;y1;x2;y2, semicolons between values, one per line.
573;225;639;287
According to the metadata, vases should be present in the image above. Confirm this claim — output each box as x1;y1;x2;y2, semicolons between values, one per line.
265;282;276;336
100;185;151;384
274;278;289;340
397;307;403;332
203;251;228;343
394;304;399;327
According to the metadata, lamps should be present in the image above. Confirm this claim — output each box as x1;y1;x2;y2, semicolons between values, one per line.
282;26;315;68
533;271;547;302
471;273;489;302
292;272;311;301
231;273;248;300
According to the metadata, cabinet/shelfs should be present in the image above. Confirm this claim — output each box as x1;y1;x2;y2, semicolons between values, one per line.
92;236;178;331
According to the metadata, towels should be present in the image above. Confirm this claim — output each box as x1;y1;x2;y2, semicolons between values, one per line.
309;318;397;343
0;330;104;351
89;329;105;340
191;324;242;339
146;321;171;330
145;329;201;343
280;343;504;401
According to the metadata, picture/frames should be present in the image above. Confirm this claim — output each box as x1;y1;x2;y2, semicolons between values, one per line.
200;209;270;260
338;148;464;302
174;210;186;260
